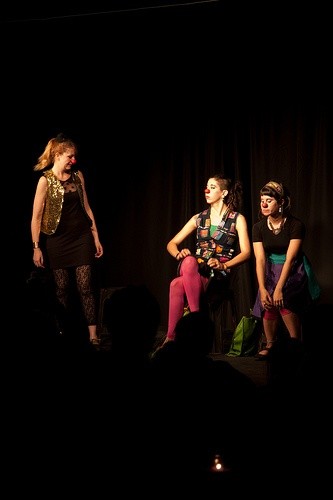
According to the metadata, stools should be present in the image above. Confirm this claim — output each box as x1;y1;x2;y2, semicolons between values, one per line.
202;290;237;357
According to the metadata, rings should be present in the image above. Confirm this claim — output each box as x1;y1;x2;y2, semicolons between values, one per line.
264;308;266;310
215;263;216;266
264;304;267;308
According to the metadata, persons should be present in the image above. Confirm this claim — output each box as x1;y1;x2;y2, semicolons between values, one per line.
31;137;104;344
152;174;251;357
251;181;320;358
0;284;333;500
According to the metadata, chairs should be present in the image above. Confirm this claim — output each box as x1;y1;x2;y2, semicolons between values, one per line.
96;286;126;339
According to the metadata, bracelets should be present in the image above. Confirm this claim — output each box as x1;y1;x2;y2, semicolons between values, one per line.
175;252;180;259
223;263;227;267
32;242;40;248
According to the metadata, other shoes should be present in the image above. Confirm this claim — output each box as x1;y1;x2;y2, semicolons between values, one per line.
90;338;100;345
259;343;273;355
